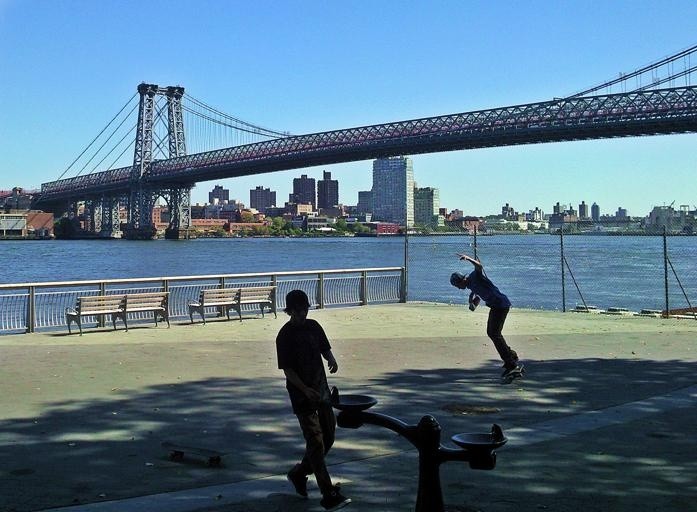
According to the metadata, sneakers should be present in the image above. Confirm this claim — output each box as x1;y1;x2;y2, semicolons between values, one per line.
322;487;352;512
501;363;520;377
288;463;309;499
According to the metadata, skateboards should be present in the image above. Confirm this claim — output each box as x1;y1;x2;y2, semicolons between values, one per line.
502;364;526;385
159;441;230;463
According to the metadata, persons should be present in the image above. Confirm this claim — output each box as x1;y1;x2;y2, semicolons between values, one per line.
449;251;519;377
275;289;352;510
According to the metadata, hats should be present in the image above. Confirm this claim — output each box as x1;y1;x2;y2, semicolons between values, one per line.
285;289;310;309
450;271;468;286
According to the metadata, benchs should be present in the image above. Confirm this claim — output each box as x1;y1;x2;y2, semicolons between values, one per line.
65;285;277;336
